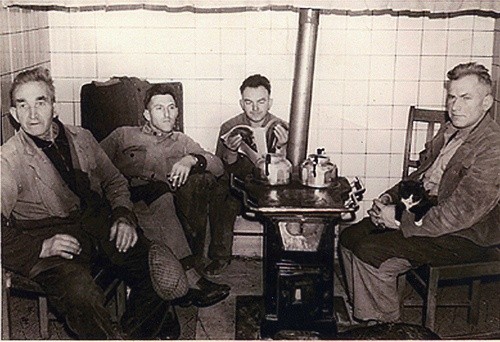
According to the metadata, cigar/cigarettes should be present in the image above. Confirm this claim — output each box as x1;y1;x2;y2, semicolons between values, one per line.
167;176;178;180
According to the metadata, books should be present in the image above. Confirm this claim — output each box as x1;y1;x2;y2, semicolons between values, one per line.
221;119;284;157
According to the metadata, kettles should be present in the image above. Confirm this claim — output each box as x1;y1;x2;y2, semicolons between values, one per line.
252;148;292;185
299;148;338;189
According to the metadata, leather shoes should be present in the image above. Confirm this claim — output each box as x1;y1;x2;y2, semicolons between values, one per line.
171;277;231;308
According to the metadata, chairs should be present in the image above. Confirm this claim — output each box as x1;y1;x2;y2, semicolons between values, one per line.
1;82;209;340
400;106;500;340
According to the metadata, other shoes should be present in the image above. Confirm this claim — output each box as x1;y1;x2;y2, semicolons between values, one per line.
204;259;228;274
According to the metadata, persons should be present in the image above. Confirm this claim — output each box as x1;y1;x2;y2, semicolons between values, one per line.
99;84;231;340
0;67;171;340
338;63;500;332
204;75;290;275
